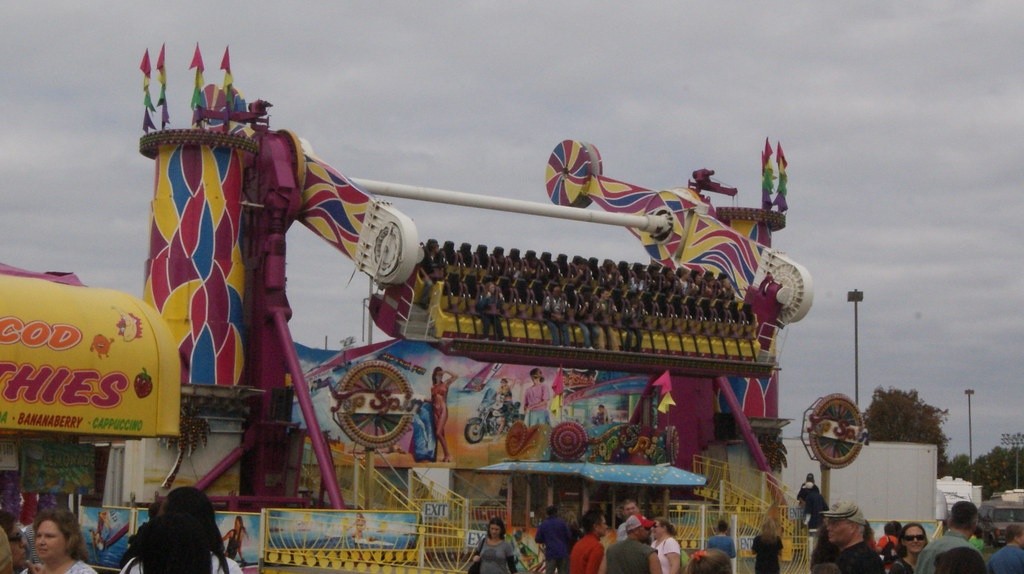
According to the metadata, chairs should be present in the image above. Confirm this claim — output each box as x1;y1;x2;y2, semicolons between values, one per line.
414;265;760;362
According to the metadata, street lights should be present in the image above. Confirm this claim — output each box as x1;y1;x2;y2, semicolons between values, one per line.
846;288;864;408
964;389;975;466
1001;432;1024;489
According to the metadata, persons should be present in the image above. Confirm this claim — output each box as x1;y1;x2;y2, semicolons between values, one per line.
0;486;243;574
796;473;1024;574
535;499;736;574
427;239;735;352
752;518;783;574
222;516;248;564
472;517;518;574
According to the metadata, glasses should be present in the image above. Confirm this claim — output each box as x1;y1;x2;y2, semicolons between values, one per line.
7;528;24;543
905;535;925;541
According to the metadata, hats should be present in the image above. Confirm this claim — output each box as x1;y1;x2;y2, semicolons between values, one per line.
625;513;655;531
820;504;866;525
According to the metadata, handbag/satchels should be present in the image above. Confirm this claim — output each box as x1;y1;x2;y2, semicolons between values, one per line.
467;557;482;574
882;541;897;564
